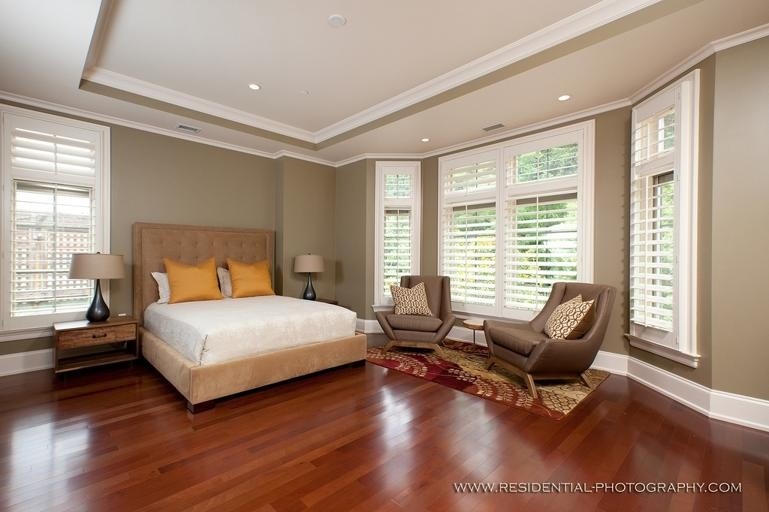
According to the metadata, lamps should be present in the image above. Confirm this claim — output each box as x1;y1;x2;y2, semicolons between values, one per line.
294;253;325;300
68;252;126;323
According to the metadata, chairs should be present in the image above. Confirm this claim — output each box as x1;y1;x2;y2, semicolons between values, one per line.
371;276;456;354
483;282;618;398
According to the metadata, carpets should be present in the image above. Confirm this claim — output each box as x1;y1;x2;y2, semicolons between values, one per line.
367;338;610;420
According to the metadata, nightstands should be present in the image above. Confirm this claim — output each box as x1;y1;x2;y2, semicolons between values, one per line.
317;299;337;305
53;315;139;374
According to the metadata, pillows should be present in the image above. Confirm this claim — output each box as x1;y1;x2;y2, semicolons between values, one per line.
163;256;225;304
217;266;232;298
544;294;595;340
227;258;276;299
390;282;433;316
152;272;170;303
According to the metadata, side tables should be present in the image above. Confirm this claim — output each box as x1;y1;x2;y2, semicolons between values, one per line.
464;319;483;351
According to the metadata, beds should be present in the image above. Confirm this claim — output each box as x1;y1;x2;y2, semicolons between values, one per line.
133;222;366;412
